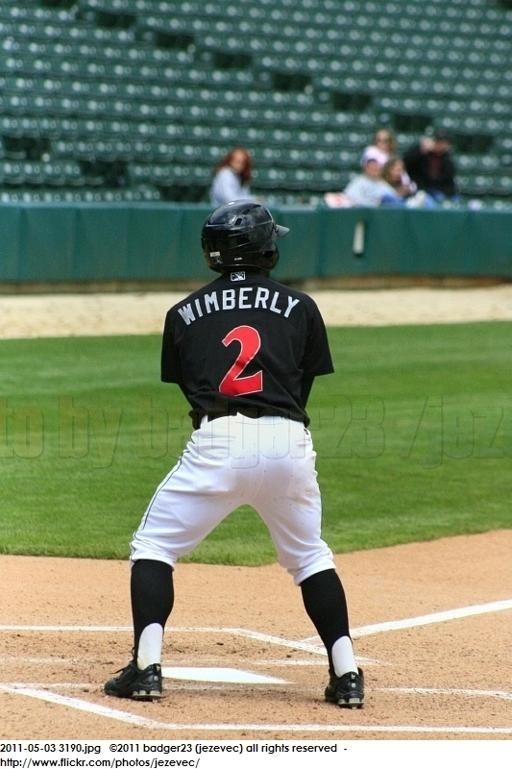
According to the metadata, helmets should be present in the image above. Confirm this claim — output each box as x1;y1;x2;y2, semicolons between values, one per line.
201;200;291;273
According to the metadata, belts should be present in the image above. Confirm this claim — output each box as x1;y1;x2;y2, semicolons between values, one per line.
194;409;236;430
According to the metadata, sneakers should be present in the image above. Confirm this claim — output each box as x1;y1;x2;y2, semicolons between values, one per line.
104;661;163;700
324;666;364;708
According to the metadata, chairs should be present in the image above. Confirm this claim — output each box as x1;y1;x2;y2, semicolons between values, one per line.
0;2;512;210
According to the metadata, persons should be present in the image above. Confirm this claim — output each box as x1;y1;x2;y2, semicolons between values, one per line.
100;198;364;711
338;123;458;210
206;145;252;204
